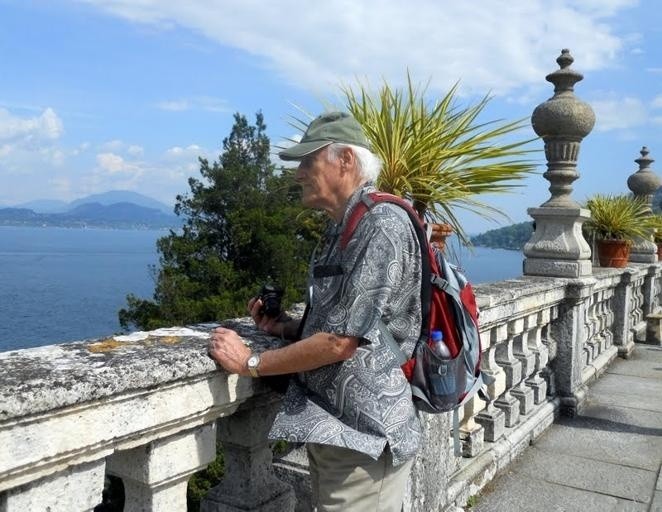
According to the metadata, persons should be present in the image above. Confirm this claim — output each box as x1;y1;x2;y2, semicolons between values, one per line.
208;109;425;512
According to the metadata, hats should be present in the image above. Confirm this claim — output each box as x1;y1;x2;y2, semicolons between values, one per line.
279;111;370;162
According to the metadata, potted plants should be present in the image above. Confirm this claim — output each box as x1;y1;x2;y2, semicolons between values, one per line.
580;192;662;268
266;79;548;255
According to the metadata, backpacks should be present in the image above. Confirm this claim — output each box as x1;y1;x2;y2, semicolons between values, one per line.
339;192;491;459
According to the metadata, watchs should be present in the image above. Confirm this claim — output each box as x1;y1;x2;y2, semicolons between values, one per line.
246;352;261;379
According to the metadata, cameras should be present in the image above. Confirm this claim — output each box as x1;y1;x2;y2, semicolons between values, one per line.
255;281;285;319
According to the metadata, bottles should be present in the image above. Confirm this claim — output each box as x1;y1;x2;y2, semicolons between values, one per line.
427;329;458;412
